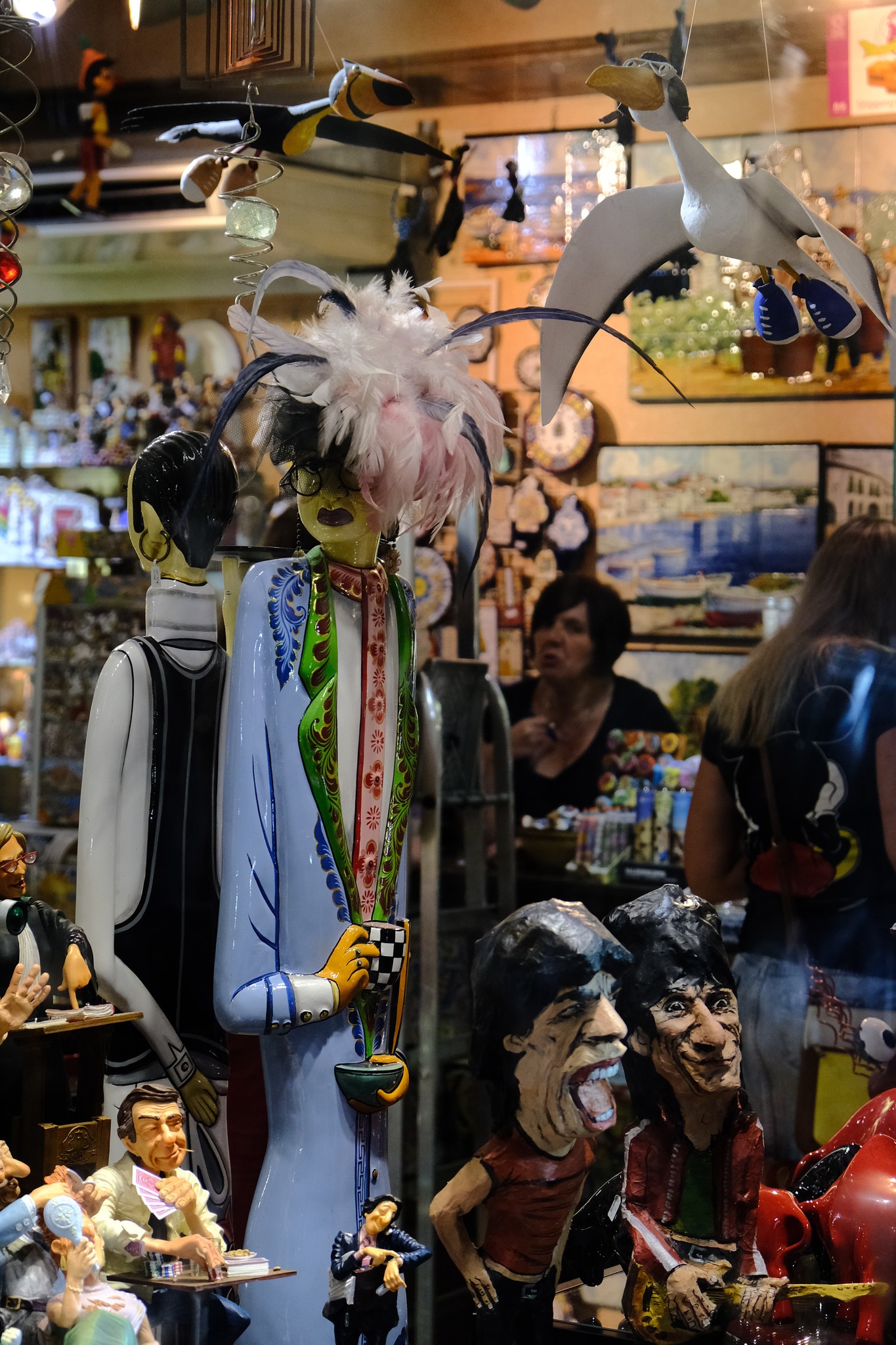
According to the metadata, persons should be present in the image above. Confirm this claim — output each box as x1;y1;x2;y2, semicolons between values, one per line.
204;310;429;1345
58;32;135;220
605;883;791;1345
0;816;254;1345
429;897;639;1345
322;1193;433;1345
70;429;236;1157
472;570;684;844
681;514;896;1191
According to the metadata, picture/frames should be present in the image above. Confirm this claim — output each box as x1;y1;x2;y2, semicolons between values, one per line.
596;439;896;646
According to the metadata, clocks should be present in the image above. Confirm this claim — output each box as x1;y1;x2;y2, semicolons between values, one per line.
515;346;540;391
523;388;594;472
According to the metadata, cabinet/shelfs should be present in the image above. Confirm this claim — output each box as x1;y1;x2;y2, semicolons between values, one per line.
0;461;130;766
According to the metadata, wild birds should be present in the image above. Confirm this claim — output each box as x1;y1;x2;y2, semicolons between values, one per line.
535;44;896;427
119;56;457;209
